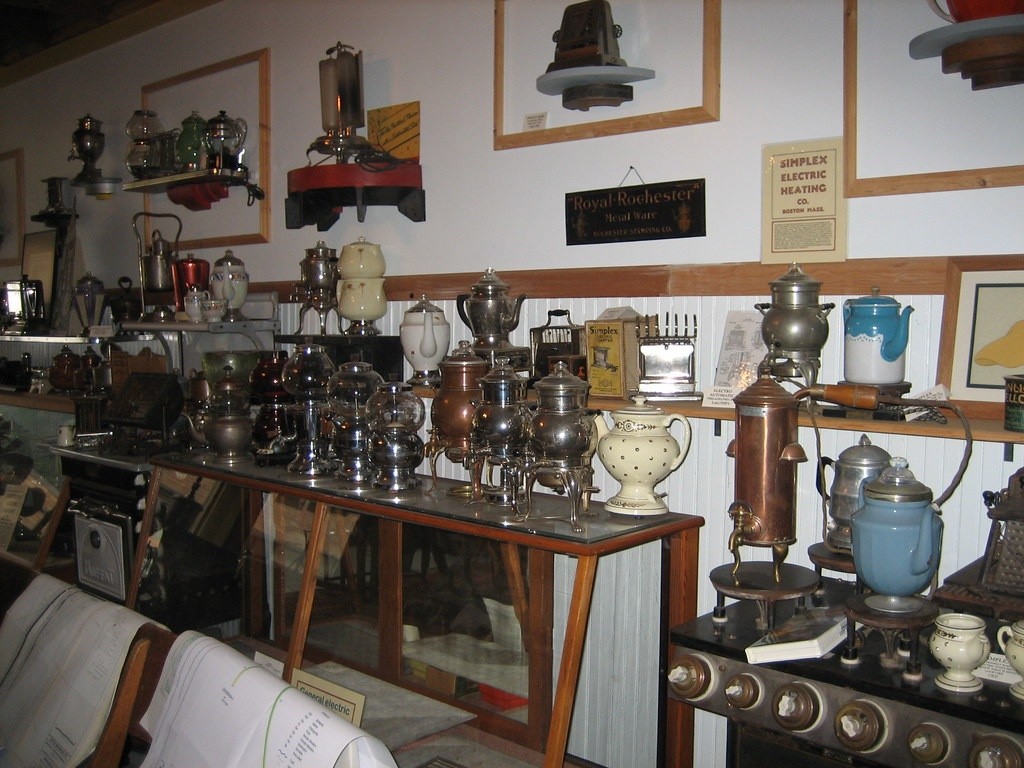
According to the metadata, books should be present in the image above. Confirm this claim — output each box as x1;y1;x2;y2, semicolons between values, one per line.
744;605;863;664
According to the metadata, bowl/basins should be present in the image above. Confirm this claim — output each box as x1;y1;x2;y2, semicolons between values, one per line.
200;300;229;322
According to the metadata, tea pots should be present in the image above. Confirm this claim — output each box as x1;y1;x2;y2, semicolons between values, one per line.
181;407;253;464
816;434;892;548
400;294;450;386
210;250;249;322
843;286;915;384
457;267;527;350
851;457;944;613
205;111;247;168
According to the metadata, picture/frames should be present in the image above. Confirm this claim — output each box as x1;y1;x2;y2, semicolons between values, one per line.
141;47;271;253
934;253;1024;421
0;148;27;266
842;1;1024;199
493;0;722;151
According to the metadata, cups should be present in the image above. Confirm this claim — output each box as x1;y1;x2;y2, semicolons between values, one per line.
997;621;1024;699
930;613;990;692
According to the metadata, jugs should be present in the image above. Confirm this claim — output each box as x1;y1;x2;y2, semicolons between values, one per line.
595;396;691;515
184;287;210;323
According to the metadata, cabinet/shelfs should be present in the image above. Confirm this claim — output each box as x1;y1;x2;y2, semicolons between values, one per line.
0;388;707;768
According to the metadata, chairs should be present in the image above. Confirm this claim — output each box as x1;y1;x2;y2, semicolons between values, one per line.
83;636;151;767
